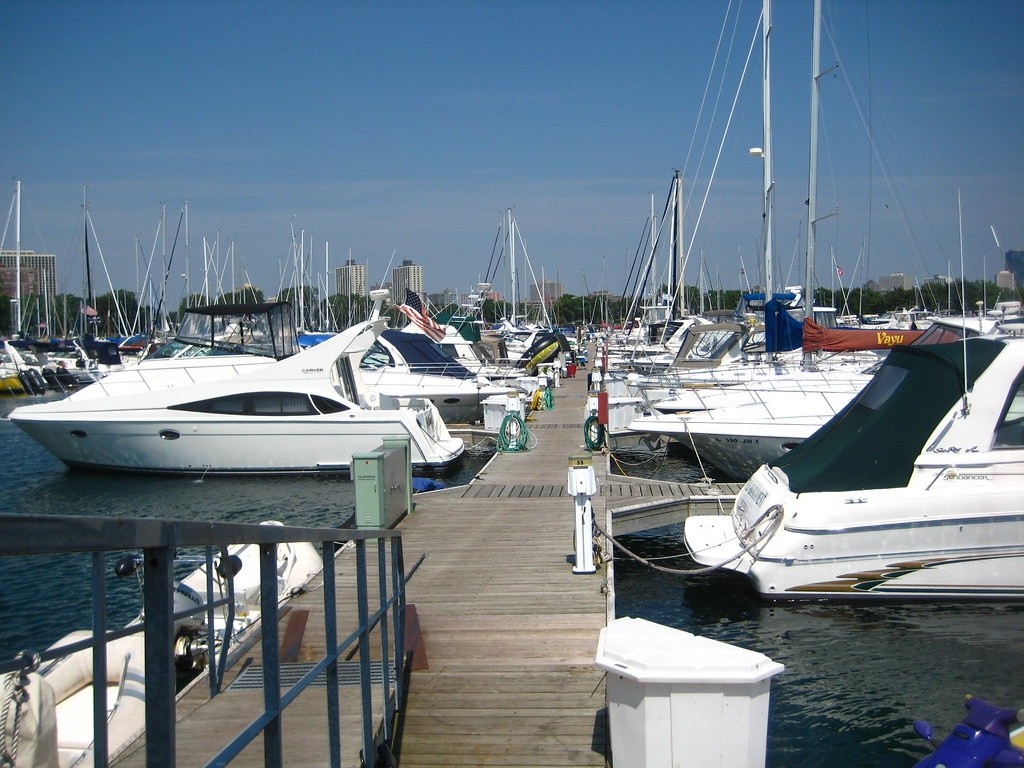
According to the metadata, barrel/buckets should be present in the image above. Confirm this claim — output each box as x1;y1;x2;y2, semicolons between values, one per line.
567;365;576;378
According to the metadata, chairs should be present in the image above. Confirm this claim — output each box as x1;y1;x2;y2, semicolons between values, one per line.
253;398;310;414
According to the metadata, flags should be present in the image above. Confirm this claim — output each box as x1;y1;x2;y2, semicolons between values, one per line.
394;287;447;342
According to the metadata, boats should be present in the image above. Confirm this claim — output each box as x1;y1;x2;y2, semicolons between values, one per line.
0;520;323;768
586;0;1024;485
0;181;579;423
8;356;464;475
685;334;1024;601
